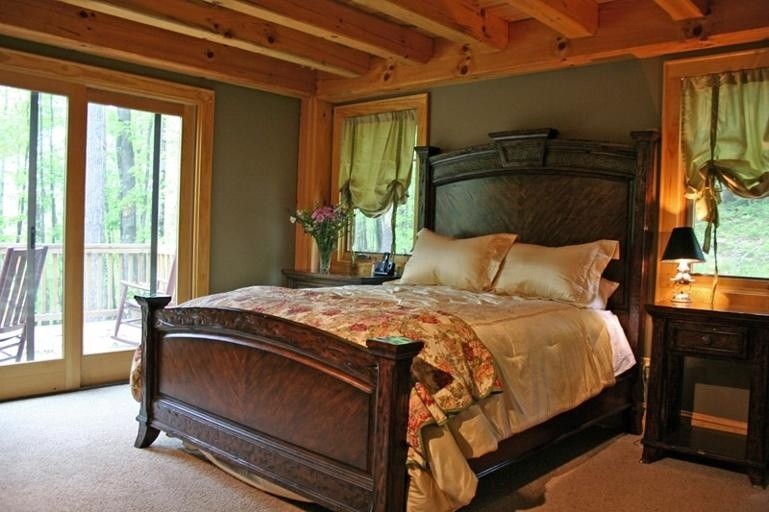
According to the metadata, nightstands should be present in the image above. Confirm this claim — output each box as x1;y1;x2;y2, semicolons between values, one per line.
639;298;769;489
280;264;393;288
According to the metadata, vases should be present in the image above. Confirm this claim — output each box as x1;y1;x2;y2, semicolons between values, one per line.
318;251;332;275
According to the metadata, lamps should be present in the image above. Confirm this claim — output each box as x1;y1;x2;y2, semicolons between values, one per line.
660;226;707;302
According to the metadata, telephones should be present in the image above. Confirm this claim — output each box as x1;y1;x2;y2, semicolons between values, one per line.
374;252;395;276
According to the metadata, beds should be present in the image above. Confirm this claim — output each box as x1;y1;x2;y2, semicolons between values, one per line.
132;126;662;512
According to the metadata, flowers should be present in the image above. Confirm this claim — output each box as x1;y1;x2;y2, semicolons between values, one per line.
286;197;356;272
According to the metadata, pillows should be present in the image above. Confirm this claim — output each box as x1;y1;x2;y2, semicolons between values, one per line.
398;228;619;309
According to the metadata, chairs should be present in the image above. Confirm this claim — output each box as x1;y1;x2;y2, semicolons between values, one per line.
111;255;176;348
0;245;49;362
